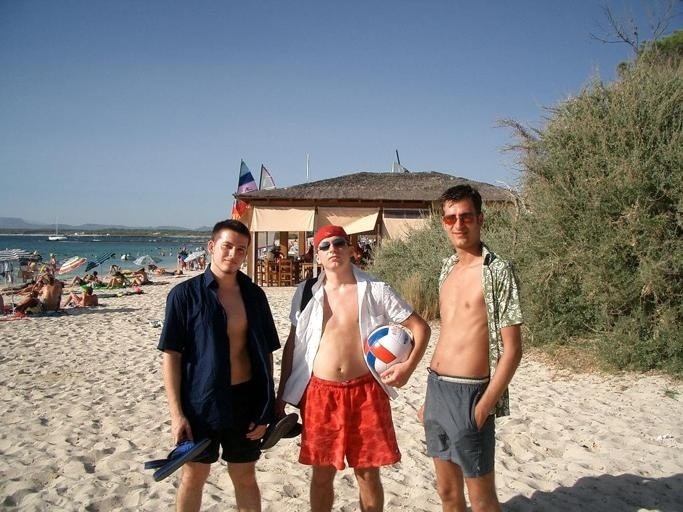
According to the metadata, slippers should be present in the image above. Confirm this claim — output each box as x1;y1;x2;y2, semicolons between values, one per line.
145;441;208;469
258;413;302;449
153;438;212;482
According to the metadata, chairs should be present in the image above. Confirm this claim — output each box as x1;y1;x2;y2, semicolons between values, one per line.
256;255;306;287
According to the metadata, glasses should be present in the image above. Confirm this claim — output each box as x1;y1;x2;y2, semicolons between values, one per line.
319;239;349;250
441;213;478;225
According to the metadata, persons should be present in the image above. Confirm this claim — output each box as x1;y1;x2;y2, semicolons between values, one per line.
157;219;281;512
416;186;524;512
264;243;373;272
0;246;208;315
274;227;432;512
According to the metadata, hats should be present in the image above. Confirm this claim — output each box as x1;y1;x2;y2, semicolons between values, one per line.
314;226;349;248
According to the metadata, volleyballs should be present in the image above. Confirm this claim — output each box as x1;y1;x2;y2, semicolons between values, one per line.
363;324;415;377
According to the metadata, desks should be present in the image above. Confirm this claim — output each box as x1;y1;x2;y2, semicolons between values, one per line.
302;263;324;281
